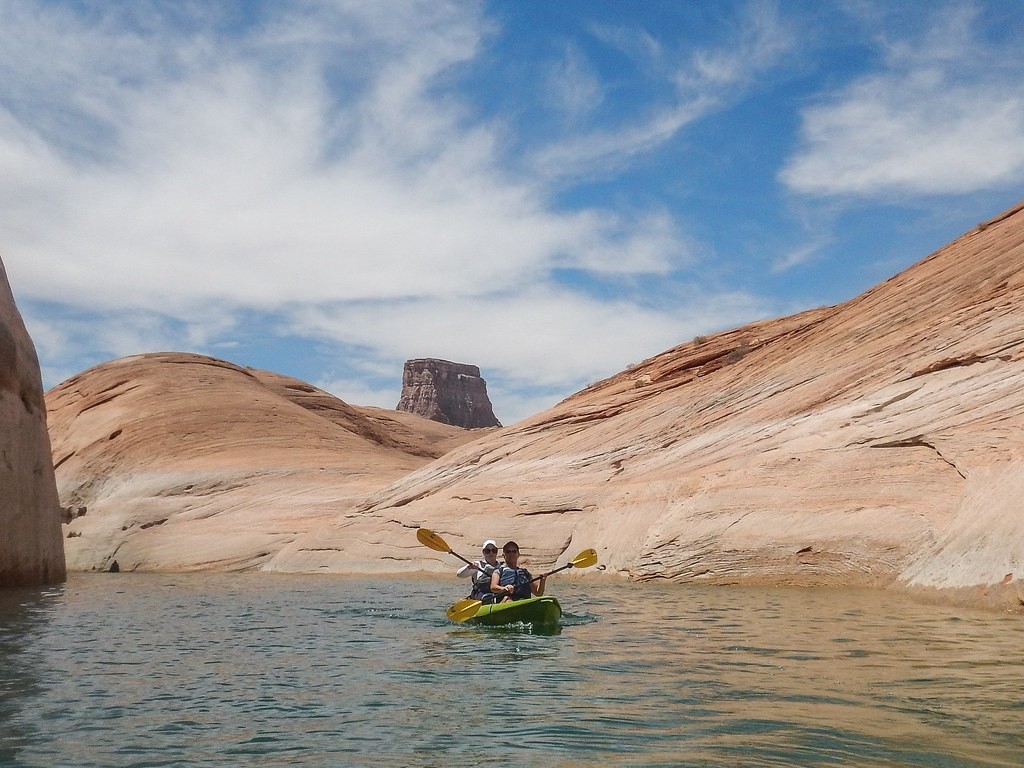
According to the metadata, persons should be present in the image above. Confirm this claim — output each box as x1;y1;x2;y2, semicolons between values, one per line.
457;540;501;604
490;541;547;604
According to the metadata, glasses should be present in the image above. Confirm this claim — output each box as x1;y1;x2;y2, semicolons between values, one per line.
485;548;498;554
504;550;518;554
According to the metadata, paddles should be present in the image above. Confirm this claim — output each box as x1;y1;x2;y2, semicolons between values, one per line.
445;547;598;623
417;527;493;578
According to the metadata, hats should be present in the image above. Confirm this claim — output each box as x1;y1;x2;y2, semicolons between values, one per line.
502;540;519;553
482;540;499;553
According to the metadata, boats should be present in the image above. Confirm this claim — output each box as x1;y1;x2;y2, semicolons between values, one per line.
446;598;563;627
